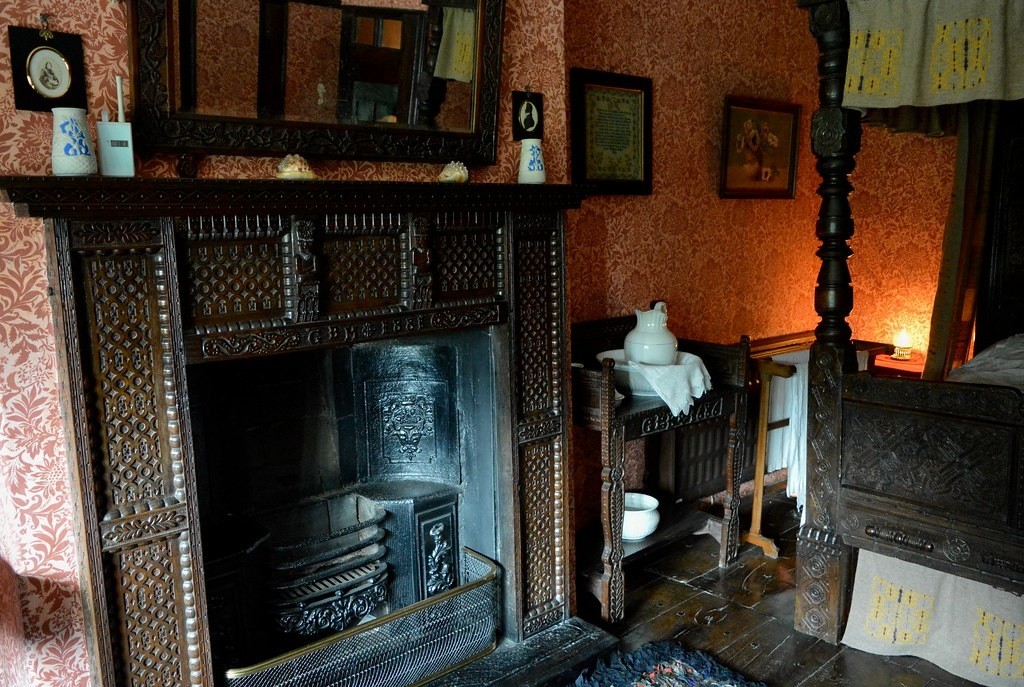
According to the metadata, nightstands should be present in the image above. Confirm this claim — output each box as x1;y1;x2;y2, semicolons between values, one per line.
868;349;928;380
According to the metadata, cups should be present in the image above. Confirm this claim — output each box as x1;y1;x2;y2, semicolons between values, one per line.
51;107;98;177
518;138;546;184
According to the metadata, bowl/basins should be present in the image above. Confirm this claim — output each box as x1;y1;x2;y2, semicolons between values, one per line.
621;492;660;544
597;349;662;397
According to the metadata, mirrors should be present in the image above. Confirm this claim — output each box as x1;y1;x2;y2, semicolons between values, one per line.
122;0;510;166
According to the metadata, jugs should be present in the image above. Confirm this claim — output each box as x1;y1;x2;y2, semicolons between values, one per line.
624;301;678;366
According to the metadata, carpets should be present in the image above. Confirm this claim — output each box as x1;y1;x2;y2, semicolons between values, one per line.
574;639;771;687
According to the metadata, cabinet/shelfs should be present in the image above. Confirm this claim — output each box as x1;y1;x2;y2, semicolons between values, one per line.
567;297;751;626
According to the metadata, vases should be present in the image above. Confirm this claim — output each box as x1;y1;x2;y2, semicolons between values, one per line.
517;138;546;184
52;108;98;178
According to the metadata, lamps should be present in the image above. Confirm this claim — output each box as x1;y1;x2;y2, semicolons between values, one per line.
889;327;913;360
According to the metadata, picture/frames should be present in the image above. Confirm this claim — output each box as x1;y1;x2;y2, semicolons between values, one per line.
568;66;653;195
717;93;803;200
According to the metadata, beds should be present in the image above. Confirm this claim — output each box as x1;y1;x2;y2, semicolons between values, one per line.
791;1;1024;687
